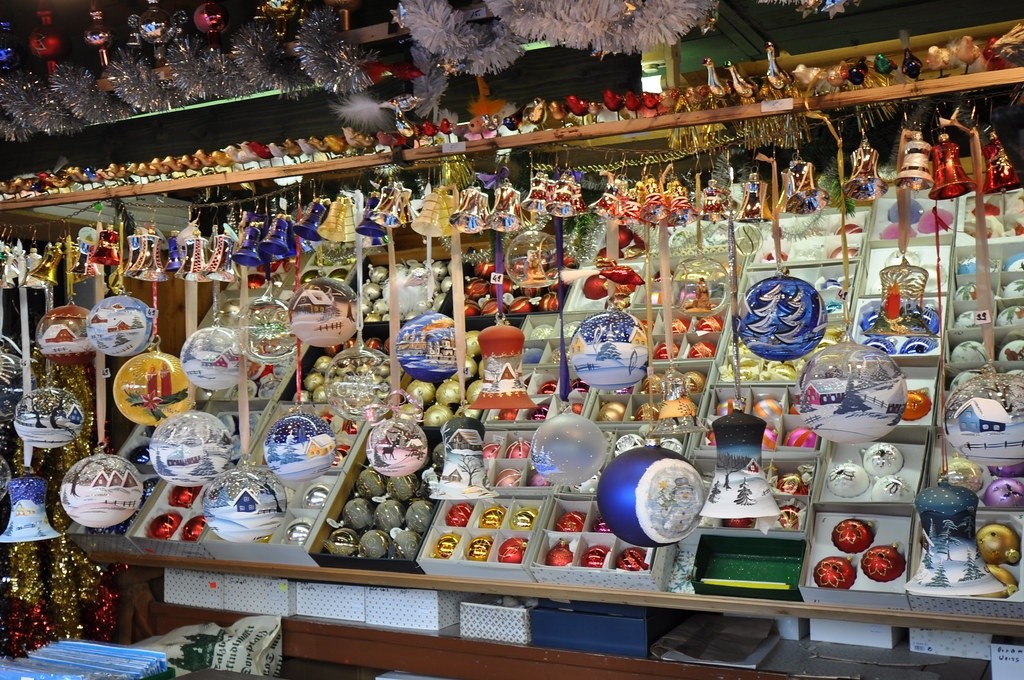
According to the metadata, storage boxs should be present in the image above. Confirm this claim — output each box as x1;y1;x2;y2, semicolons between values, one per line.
66;185;1024;680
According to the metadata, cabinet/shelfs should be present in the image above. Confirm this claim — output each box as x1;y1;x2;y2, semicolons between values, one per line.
84;549;1024;680
